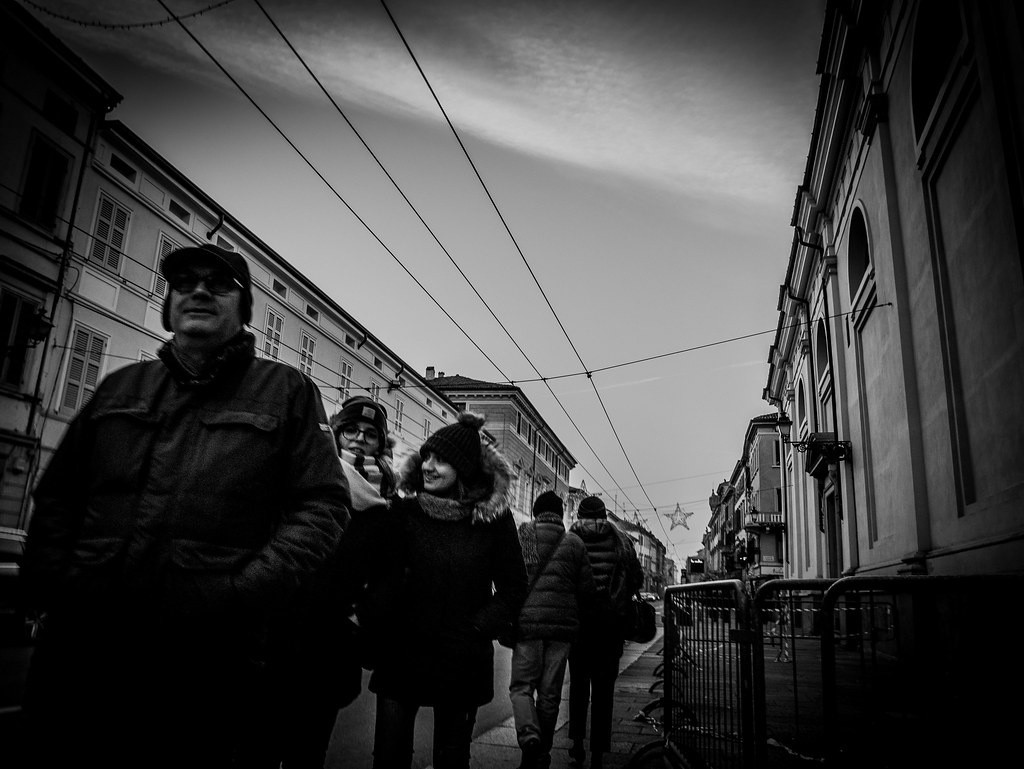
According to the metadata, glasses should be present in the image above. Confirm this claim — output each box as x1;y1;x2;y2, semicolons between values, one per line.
342;425;378;445
170;262;243;294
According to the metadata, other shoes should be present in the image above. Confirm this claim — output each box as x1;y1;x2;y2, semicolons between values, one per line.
568;744;601;766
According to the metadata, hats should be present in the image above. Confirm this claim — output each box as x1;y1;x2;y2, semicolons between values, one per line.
420;413;486;479
534;491;563;517
162;243;253;333
334;395;387;452
579;496;606;520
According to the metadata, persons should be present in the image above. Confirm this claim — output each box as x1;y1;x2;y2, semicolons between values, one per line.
500;488;595;767
323;392;406;760
358;412;529;769
561;495;644;766
6;241;356;767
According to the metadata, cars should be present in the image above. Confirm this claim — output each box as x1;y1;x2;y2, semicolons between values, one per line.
632;591;661;603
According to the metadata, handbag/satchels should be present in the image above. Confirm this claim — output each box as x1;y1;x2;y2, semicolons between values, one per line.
627;588;655;645
